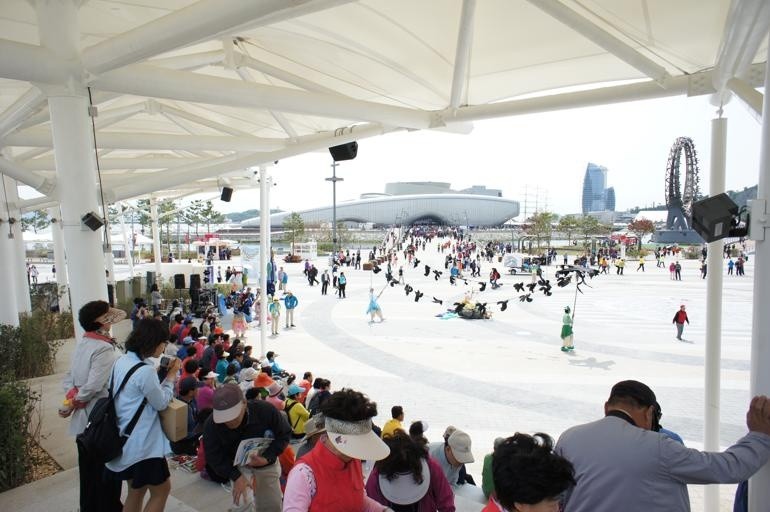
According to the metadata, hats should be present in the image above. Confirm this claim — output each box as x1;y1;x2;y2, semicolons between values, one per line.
268;381;284;397
286;385;306;397
412;418;430;433
238;368;260;382
219;351;230;357
254;372;273;387
197;336;208;339
180;376;205;388
184;320;194;324
611;380;656;405
202;370;220;379
313;411;391;461
443;426;455;438
378;456;431;506
94;306;128;326
448;430;475;464
183;336;196;345
301;412;326;444
212;384;244;425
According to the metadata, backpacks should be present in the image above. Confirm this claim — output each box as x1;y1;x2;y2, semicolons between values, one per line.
281;400;301;435
76;362;148;465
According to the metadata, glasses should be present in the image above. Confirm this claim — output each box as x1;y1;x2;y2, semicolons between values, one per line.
163;340;169;347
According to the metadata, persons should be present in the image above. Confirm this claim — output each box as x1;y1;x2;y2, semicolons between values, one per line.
170;224;748;298
60;283;768;512
